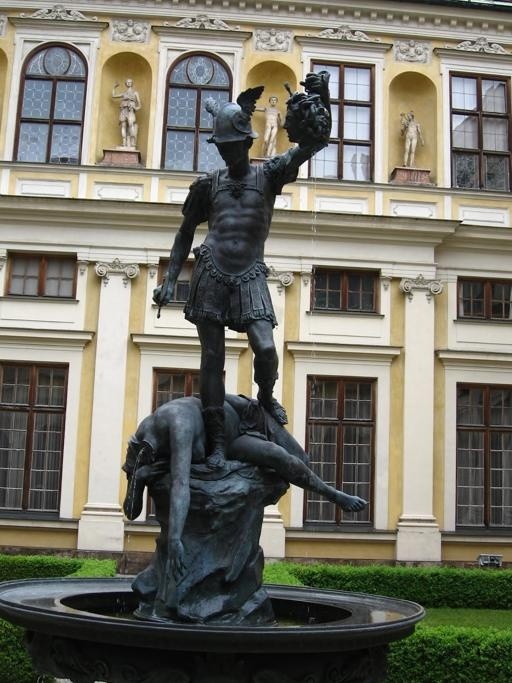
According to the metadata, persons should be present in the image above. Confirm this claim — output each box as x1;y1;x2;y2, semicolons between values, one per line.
120;392;369;582
153;72;331;468
253;96;284;159
400;110;424;168
282;89;330;144
112;77;142;149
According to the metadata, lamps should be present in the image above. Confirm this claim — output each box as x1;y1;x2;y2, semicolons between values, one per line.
475;554;504;568
